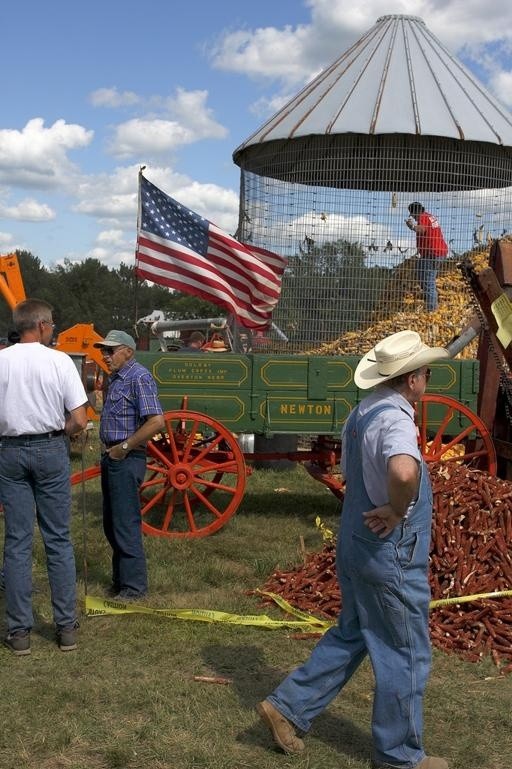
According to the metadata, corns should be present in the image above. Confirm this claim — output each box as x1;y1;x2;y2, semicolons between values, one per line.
392;193;396;207
300;228;511;359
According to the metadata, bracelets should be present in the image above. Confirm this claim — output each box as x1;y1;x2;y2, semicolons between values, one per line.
410;223;415;231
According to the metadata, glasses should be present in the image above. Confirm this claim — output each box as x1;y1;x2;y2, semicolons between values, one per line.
44;320;56;329
100;347;127;355
413;369;431;383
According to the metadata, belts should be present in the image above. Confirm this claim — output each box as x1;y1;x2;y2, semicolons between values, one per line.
2;431;64;442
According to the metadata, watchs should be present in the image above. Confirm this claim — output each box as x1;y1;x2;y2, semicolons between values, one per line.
121;440;132;452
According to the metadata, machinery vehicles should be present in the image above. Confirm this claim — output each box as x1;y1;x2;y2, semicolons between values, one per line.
0;256;113;427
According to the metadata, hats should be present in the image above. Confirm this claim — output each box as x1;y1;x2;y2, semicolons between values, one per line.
94;330;136;351
354;330;451;389
205;340;228;352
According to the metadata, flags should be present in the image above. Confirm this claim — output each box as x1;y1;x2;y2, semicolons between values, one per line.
134;174;289;335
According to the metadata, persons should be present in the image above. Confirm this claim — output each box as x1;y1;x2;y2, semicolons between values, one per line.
174;328;275;354
94;330;166;605
0;299;89;655
404;202;450;313
256;330;453;768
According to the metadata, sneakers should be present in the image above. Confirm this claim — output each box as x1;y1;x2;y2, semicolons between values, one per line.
255;700;304;754
416;756;448;769
56;628;78;651
4;633;32;655
